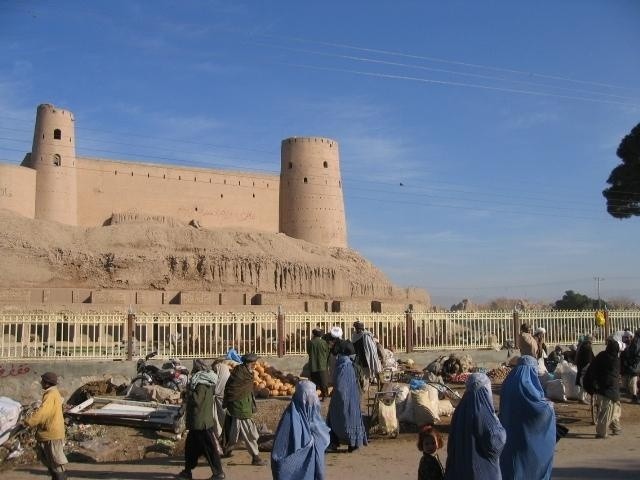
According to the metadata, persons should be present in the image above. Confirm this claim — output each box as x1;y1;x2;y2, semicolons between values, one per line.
173;359;225;480
211;363;231;457
221;353;269;465
25;372;68;480
271;380;330;480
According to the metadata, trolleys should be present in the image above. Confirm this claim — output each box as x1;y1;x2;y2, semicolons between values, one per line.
427;381;461;411
369;391;399;441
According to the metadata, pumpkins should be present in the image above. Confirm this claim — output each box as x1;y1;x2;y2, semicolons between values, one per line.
253;362;295;396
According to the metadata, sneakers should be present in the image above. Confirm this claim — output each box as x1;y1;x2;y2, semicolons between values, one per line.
209;473;225;480
177;470;192;480
252;457;269;466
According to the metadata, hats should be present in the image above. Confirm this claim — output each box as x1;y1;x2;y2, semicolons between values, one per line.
312;328;323;337
354;320;365;330
331;327;343;338
41;372;58;385
536;327;546;335
241;353;258;362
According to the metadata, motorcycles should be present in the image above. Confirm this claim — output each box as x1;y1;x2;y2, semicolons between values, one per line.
131;351;189;393
